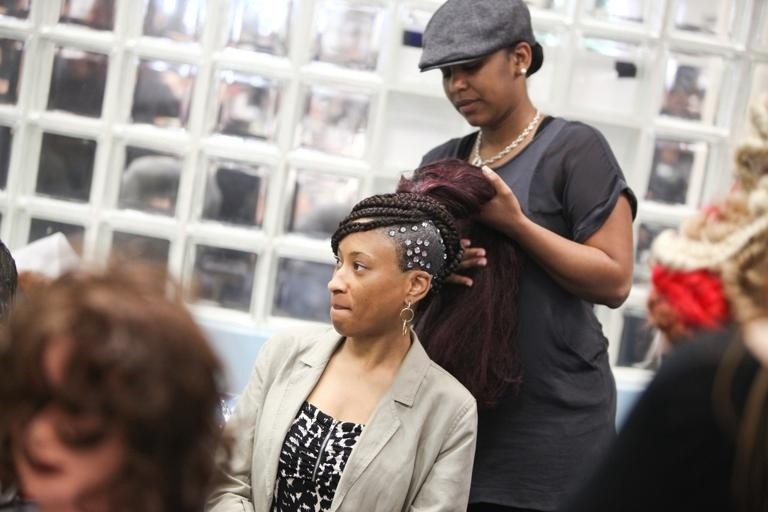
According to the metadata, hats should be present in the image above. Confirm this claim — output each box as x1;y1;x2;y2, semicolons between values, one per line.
419;0;537;73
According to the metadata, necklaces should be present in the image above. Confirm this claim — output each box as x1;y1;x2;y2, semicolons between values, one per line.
471;107;542;167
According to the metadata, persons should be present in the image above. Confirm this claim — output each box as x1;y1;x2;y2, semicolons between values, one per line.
572;93;767;512
201;154;523;511
409;0;637;512
1;255;226;512
